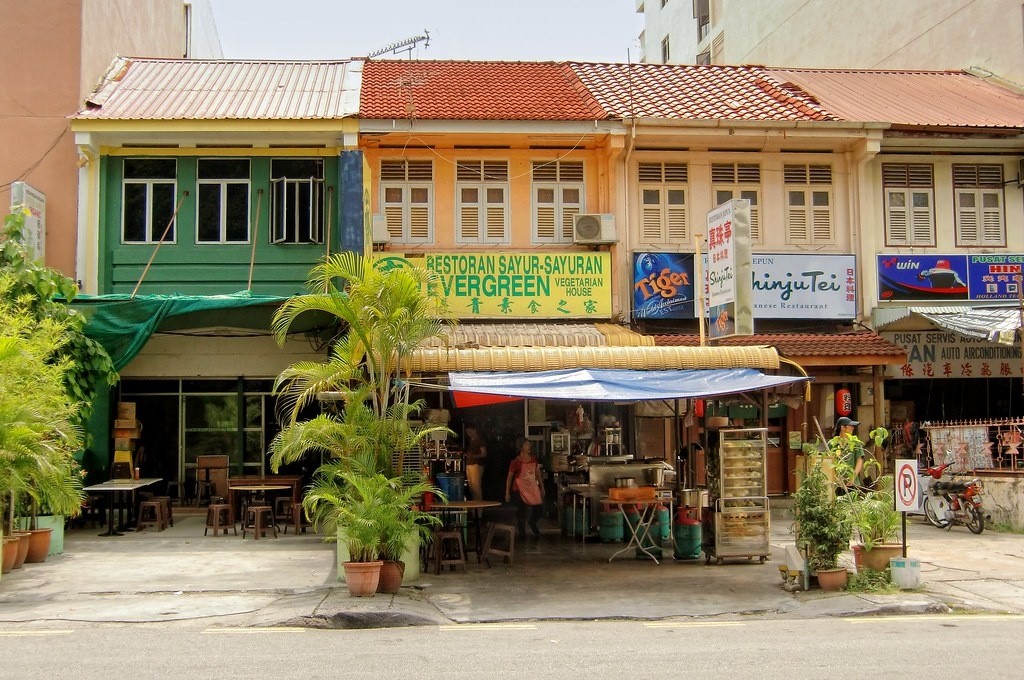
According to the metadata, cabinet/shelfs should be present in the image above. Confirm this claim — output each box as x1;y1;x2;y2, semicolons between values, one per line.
718;428;768;513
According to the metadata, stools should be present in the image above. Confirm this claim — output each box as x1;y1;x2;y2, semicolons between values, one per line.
242;496;304;539
430;531;467;576
136;480;237;541
480;522;516;567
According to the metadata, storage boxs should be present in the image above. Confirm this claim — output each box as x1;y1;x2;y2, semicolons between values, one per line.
112;403;140;479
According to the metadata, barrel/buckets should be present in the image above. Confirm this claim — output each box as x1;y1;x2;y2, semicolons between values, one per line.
437;471;464;501
642;468;662;486
681;489;697;507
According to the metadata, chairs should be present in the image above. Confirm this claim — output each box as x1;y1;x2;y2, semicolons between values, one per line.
72;461;132;532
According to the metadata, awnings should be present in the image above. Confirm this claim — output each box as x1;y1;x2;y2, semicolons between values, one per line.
353;320;817;401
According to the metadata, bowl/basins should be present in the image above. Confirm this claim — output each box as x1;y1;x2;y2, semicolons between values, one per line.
615;478;634;487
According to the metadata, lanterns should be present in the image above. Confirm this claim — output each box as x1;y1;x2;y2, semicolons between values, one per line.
837;389;852;416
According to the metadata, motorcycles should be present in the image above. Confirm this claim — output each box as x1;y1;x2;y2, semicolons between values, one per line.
920;460;986;531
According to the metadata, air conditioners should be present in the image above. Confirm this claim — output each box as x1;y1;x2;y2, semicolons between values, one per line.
572;213;617;246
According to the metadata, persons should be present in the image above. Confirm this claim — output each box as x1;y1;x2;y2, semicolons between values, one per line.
826;416;865;548
458;423;486;522
505;437;546;538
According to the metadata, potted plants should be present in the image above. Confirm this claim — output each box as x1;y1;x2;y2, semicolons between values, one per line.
264;251;461;597
789;428;910;593
0;272;92;576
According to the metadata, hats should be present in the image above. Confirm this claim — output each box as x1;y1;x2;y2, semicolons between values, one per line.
836;417;861;426
515;436;525;449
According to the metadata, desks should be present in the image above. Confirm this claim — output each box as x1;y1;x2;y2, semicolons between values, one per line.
81;478;163;536
599;496;676;565
429;500;503;568
225;477;307;535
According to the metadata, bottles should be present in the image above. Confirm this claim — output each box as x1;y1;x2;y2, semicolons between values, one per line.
608;431;618;444
135;467;139;480
